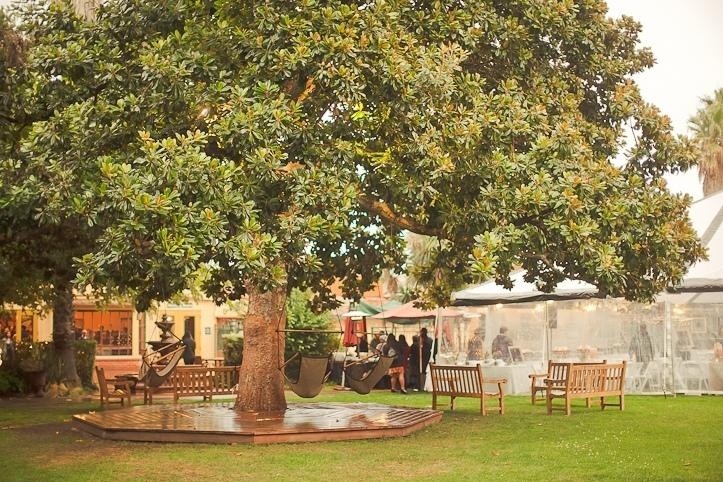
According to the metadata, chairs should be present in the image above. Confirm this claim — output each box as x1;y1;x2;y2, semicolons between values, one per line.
626;359;709;395
94;365;135;410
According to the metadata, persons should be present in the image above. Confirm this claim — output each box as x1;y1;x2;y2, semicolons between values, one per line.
81;324;132;354
492;326;513;365
181;330;196;365
629;324;695;375
0;325;29;360
370;327;433;394
466;327;489;360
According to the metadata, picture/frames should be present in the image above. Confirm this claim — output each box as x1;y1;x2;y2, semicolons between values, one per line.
508;345;524;364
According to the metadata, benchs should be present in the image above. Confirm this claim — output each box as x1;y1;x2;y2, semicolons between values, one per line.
143;364;241;405
529;360;627;415
428;361;507;415
